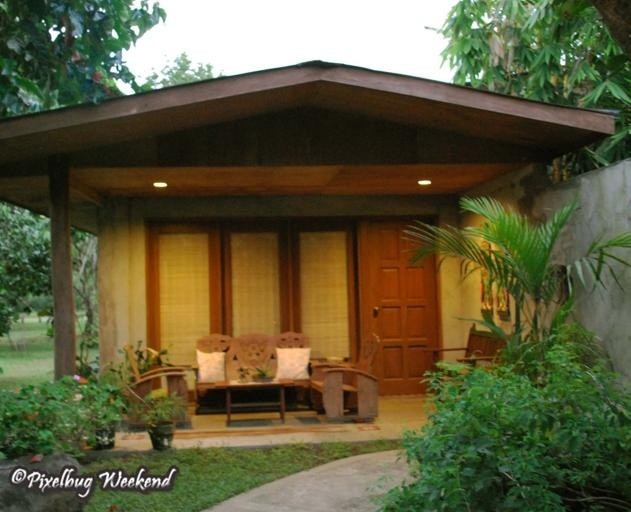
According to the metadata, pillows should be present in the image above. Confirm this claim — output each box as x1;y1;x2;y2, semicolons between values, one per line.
195;348;226;383
274;345;311;381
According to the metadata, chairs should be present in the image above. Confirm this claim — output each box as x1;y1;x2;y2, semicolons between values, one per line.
307;332;382;423
123;342;196;410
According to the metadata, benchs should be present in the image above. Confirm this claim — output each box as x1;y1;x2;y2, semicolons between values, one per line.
194;330;310;410
424;321;522;382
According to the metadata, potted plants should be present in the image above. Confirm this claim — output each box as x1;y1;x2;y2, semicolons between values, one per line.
85;387;125;452
127;390;197;451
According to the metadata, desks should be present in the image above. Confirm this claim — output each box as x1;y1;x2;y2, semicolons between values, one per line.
214;379;294;427
